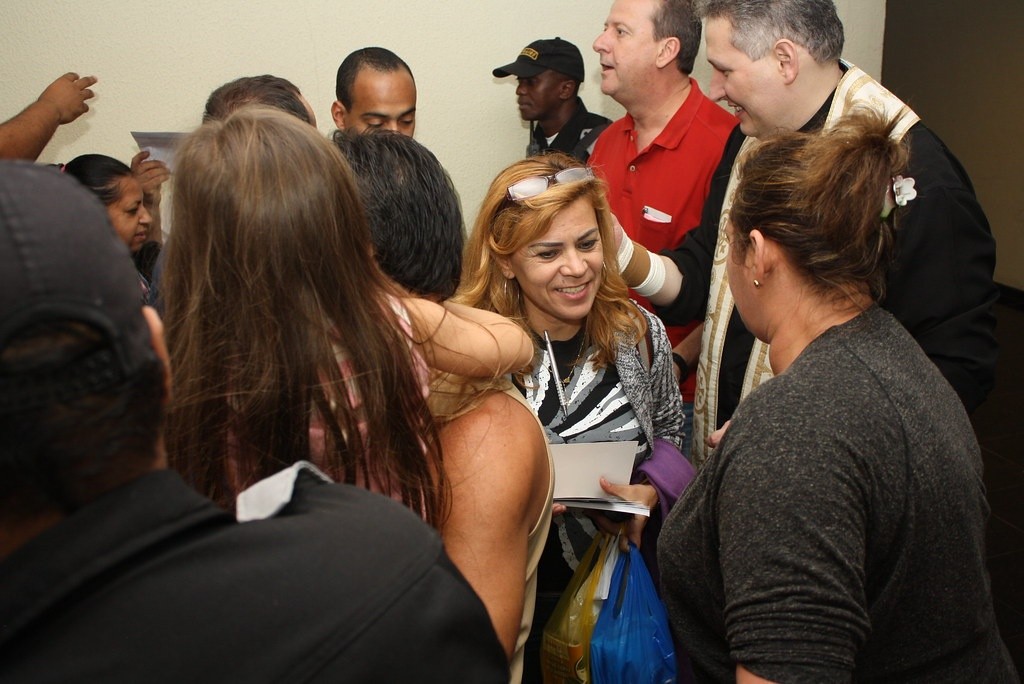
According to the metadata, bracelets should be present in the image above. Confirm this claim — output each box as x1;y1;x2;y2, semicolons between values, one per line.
672;352;690;382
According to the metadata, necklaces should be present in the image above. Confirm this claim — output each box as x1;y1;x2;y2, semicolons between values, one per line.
533;332;584;384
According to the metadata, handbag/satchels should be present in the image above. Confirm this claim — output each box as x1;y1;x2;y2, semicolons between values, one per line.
541;527;678;684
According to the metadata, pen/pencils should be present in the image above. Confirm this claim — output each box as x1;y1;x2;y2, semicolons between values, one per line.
543;328;569;417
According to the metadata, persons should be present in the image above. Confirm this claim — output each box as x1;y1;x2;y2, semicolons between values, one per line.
652;106;1017;684
453;152;687;684
202;75;318;128
330;47;415;140
1;72;170;302
607;0;996;470
492;37;612;166
586;0;739;459
0;155;513;682
159;106;534;527
327;127;554;684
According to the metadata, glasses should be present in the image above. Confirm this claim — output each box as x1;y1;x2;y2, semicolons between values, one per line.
496;166;595;217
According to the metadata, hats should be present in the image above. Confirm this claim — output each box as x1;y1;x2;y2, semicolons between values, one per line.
492;37;585;83
0;161;156;452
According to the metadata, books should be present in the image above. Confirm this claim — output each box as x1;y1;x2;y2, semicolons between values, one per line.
548;439;651;517
